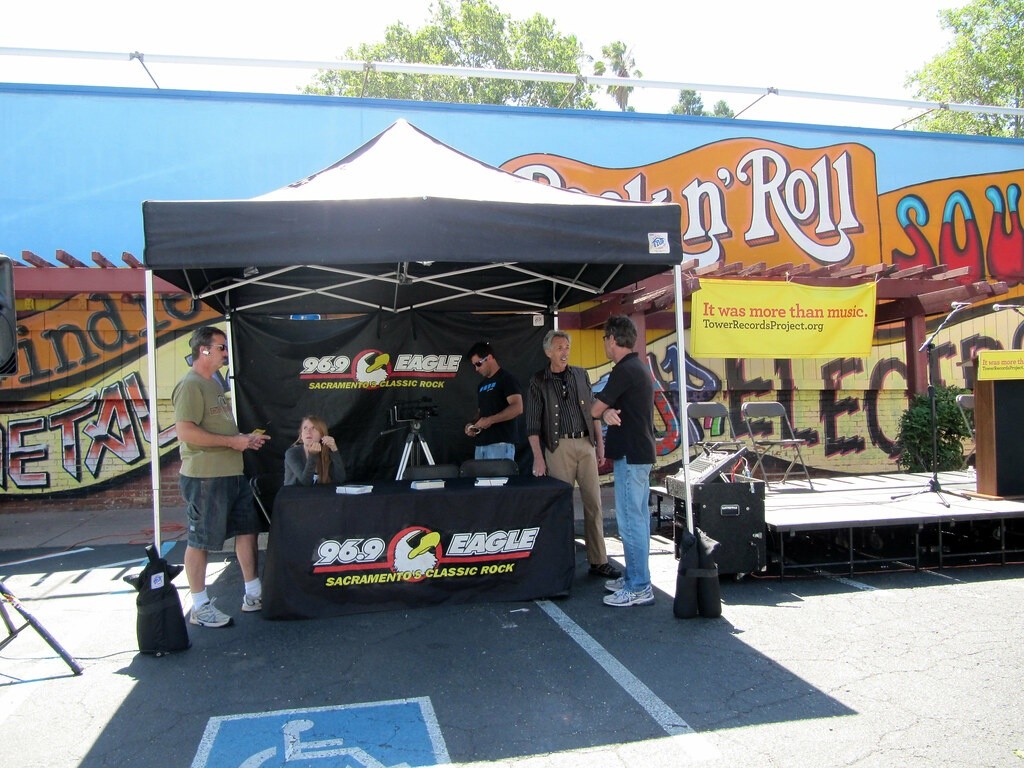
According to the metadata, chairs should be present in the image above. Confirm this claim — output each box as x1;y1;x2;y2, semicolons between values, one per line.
957;395;974;471
688;402;814;489
250;459;519;522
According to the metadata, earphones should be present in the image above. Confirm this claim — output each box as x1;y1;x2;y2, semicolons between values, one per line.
203;350;210;355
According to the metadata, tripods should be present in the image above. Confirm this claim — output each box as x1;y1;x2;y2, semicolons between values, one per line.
380;421;435;481
891;306;972;508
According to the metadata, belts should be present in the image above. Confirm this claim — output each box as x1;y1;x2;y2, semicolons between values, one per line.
559;430;589;439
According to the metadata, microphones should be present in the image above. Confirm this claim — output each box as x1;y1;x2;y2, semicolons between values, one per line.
951;301;971;309
992;304;1021;311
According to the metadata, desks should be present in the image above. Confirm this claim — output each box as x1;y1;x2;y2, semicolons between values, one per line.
274;480;574;608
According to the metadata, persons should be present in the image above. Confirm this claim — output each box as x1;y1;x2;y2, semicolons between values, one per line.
284;413;345;488
465;341;525;460
172;326;270;629
526;329;621;578
590;315;656;607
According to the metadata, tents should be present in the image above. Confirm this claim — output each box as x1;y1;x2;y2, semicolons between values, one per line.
142;118;692;556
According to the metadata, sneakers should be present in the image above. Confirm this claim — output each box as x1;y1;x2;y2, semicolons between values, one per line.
603;585;654;606
588;563;621;577
189;600;234;628
242;594;262;611
605;577;652;591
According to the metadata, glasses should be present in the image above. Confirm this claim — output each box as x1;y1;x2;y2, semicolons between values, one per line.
472;356;487;368
603;335;609;341
204;344;227;351
